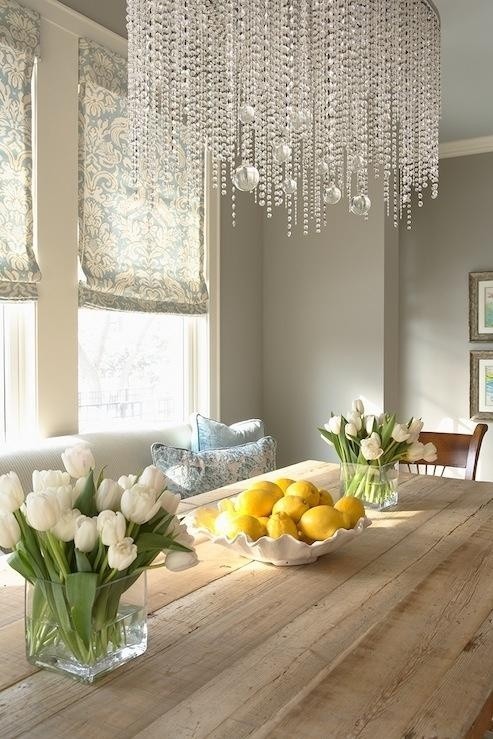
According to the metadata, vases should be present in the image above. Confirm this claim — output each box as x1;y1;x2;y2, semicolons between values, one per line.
24;573;148;685
338;463;400;511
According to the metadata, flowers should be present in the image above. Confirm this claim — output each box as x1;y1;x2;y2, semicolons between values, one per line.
2;441;202;662
316;398;440;498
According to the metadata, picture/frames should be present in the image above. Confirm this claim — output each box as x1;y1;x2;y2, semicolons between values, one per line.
467;349;493;420
468;270;493;340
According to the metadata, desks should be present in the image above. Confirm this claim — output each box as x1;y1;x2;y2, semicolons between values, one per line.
1;460;493;739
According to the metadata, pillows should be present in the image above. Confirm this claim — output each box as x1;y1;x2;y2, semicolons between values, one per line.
192;412;267;450
151;436;277;499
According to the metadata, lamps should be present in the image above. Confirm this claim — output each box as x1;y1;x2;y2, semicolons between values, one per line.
124;0;443;239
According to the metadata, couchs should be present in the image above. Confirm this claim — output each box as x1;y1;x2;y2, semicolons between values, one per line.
1;425;199;552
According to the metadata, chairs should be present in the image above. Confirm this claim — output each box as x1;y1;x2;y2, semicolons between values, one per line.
399;423;489;481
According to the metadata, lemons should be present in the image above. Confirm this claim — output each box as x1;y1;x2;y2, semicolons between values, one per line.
191;478;366;543
